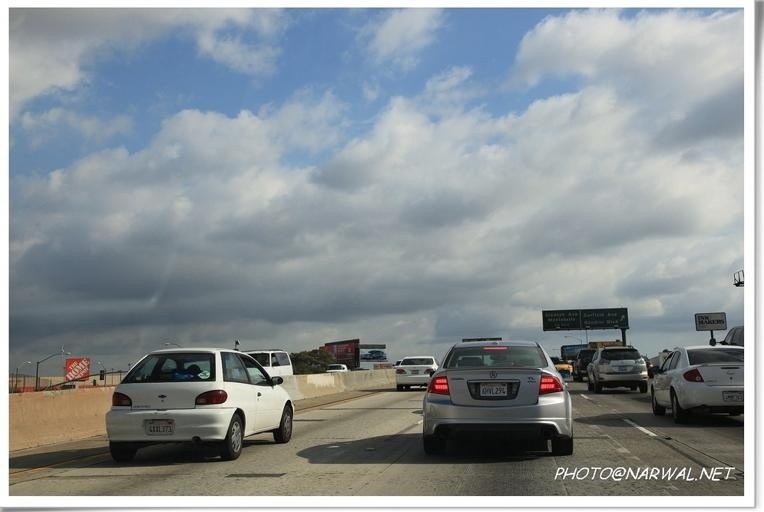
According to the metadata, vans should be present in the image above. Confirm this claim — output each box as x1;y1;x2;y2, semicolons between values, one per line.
231;349;292;381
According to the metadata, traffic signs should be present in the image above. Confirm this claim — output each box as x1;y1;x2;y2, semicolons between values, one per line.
542;308;628;331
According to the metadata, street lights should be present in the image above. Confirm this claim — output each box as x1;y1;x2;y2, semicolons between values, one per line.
165;342;182;348
35;350;71;391
233;339;240;350
14;361;31;392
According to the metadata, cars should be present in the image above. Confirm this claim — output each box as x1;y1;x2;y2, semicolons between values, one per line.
325;363;350;373
360;350;386;360
104;347;294;459
